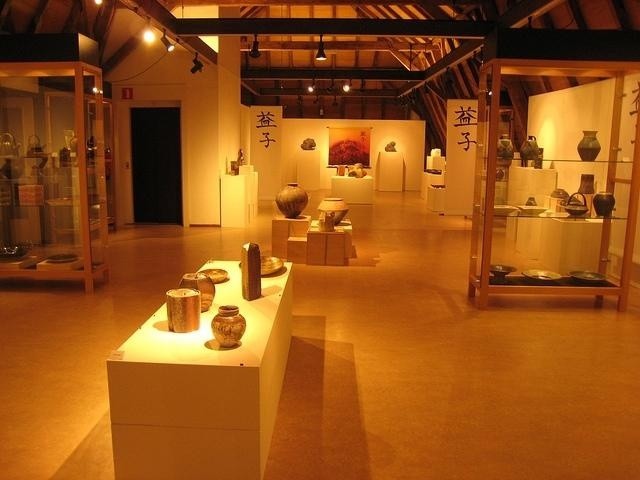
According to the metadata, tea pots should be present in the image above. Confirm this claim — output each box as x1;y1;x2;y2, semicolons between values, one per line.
27;134;47;157
0;132;22;158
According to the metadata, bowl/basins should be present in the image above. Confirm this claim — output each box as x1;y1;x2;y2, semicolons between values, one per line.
551;189;569;198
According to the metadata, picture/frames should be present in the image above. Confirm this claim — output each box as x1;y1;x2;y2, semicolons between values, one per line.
327;126;373;168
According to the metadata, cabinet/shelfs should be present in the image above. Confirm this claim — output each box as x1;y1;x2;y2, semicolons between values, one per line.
44;91;118;245
427;184;445;213
330;176;374;204
467;57;640;314
106;260;294;480
421;170;444;200
222;172;258;228
1;61;111;295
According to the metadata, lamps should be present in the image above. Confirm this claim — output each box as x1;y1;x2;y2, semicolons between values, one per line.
343;80;353;92
249;37;261;58
307;79;317;92
315;36;327;61
160;28;174;53
189;54;205;74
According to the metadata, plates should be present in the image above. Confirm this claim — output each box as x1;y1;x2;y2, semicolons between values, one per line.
47;254;80;261
475;205;519;215
521;269;562;282
568;271;608;284
0;245;28;258
239;255;284;276
198;269;228;283
515;206;549;215
